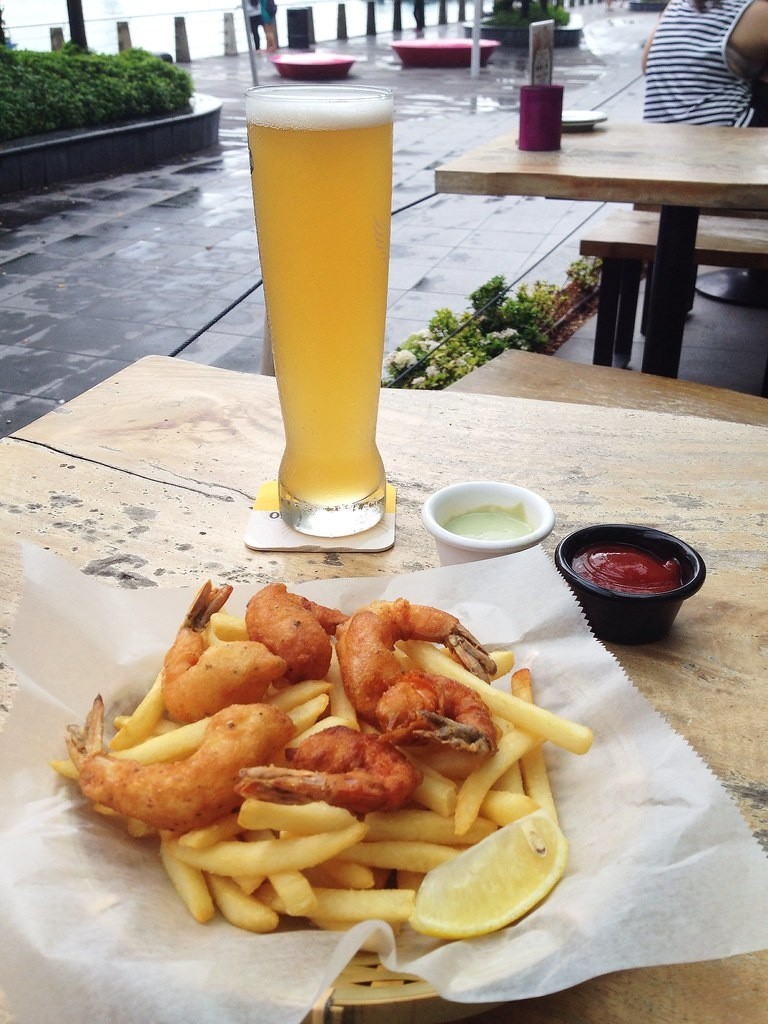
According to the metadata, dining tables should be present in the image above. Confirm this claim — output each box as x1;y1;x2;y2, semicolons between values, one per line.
0;354;768;1023
434;119;768;379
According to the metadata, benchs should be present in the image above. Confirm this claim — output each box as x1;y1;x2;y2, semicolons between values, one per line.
633;203;768;337
579;209;768;397
439;348;768;428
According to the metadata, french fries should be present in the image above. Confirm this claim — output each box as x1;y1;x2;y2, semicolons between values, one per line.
52;611;593;936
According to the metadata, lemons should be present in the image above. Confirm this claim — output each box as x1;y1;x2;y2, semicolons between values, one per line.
409;809;567;936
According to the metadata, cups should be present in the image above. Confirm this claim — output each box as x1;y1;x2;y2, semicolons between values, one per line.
245;80;395;538
518;85;564;151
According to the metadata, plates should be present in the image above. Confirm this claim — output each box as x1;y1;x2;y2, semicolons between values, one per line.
561;111;609;127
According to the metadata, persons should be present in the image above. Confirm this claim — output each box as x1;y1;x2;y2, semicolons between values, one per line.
261;0;277;51
242;0;267;53
641;0;768;131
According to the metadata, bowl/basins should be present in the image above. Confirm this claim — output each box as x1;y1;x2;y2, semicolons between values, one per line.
422;481;554;570
555;523;706;644
